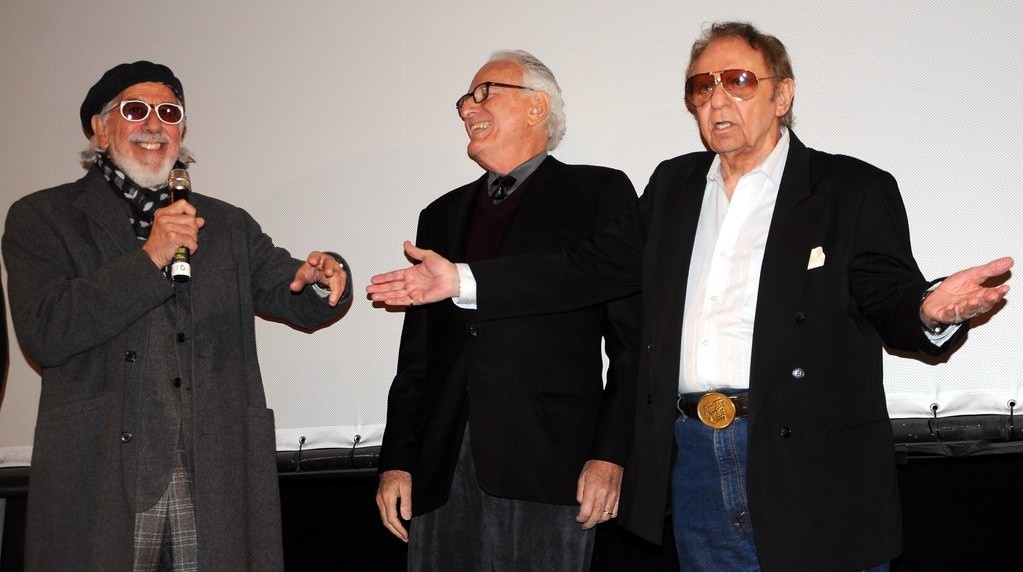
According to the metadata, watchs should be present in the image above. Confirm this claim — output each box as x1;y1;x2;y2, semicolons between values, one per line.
918;292;949;335
316;257;345;292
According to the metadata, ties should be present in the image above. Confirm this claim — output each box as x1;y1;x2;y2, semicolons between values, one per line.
489;175;518;204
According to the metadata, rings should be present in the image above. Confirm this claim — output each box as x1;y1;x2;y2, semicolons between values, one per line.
408;295;413;306
602;510;613;516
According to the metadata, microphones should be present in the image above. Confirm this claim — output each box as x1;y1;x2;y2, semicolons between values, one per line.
171;168;192;282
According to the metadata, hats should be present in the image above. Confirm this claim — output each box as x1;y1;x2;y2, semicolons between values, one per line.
80;61;185;136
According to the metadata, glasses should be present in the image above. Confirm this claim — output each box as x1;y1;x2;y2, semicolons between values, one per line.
456;81;534;119
103;100;187;138
685;68;778;109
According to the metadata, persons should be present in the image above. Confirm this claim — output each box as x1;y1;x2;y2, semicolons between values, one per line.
365;22;1013;572
376;51;637;572
0;60;351;572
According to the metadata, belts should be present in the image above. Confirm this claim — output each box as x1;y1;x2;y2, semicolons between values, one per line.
679;391;750;429
